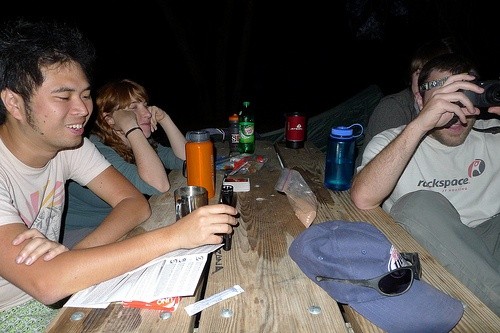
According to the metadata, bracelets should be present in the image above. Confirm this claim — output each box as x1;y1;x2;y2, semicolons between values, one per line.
124;126;144;138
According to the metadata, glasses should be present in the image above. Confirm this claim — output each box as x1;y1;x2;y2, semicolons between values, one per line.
316;252;423;296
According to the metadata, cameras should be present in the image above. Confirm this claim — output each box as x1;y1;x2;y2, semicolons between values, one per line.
462;81;500;107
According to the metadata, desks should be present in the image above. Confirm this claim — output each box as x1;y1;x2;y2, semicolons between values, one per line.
49;139;500;333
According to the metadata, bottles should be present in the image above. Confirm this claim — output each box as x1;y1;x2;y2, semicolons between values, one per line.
238;102;254;153
324;124;363;191
185;131;214;198
229;114;239;152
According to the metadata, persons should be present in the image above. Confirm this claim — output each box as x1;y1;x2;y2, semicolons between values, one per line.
350;55;500;317
58;78;186;250
353;56;430;179
0;20;238;333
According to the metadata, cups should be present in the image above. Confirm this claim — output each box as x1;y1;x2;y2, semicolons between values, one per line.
174;186;208;222
286;111;306;148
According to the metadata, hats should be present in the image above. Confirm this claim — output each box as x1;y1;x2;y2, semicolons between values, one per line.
288;221;463;333
